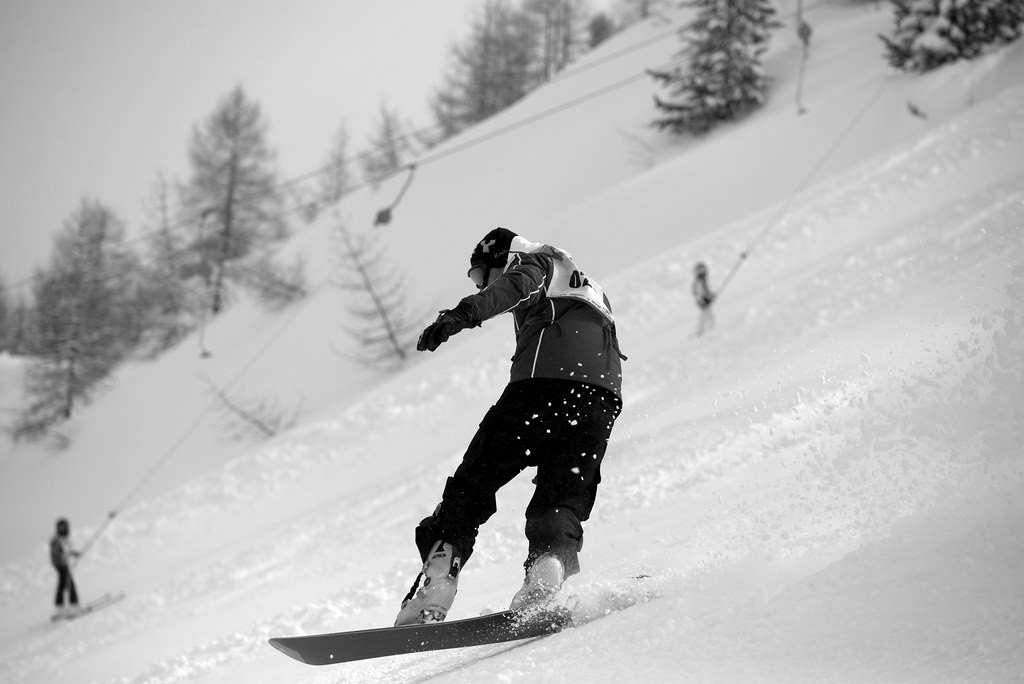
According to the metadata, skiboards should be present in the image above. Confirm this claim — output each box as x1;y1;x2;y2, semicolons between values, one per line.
51;591;125;624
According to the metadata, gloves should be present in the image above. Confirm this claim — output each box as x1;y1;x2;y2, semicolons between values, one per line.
417;301;481;352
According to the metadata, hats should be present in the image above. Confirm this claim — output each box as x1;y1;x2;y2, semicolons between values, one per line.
470;227;518;268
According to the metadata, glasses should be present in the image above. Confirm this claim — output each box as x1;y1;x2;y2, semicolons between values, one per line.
467;249;509;289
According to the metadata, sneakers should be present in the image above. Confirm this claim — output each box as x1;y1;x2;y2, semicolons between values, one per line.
395;540;462;625
509;551;565;611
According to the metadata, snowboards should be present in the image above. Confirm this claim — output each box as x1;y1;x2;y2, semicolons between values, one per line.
268;601;576;666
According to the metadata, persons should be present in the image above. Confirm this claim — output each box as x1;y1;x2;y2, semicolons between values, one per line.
691;261;716;333
50;519;80;610
393;226;623;630
